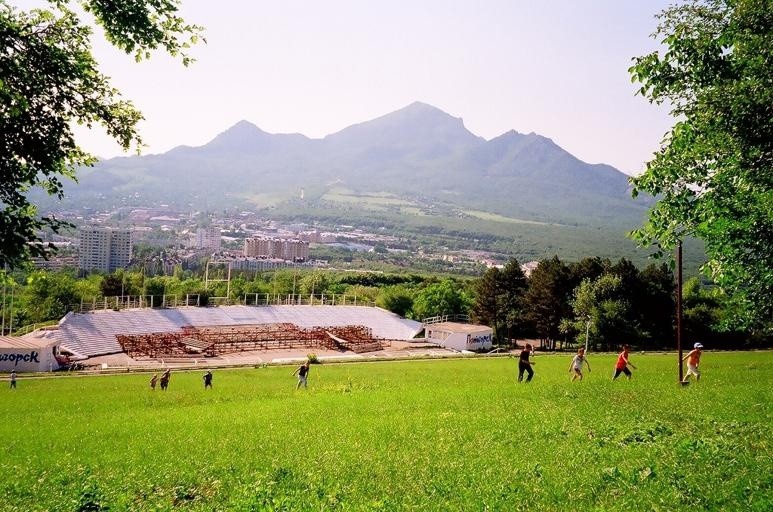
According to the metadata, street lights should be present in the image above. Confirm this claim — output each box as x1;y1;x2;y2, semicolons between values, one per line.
673;235;690;387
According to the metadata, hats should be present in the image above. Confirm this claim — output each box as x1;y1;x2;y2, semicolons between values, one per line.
694;342;704;348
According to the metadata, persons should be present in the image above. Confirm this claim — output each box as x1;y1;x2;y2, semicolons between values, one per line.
568;347;591;382
10;369;17;388
518;344;536;382
612;344;637;380
202;369;212;389
682;342;703;382
149;368;170;390
292;361;310;390
